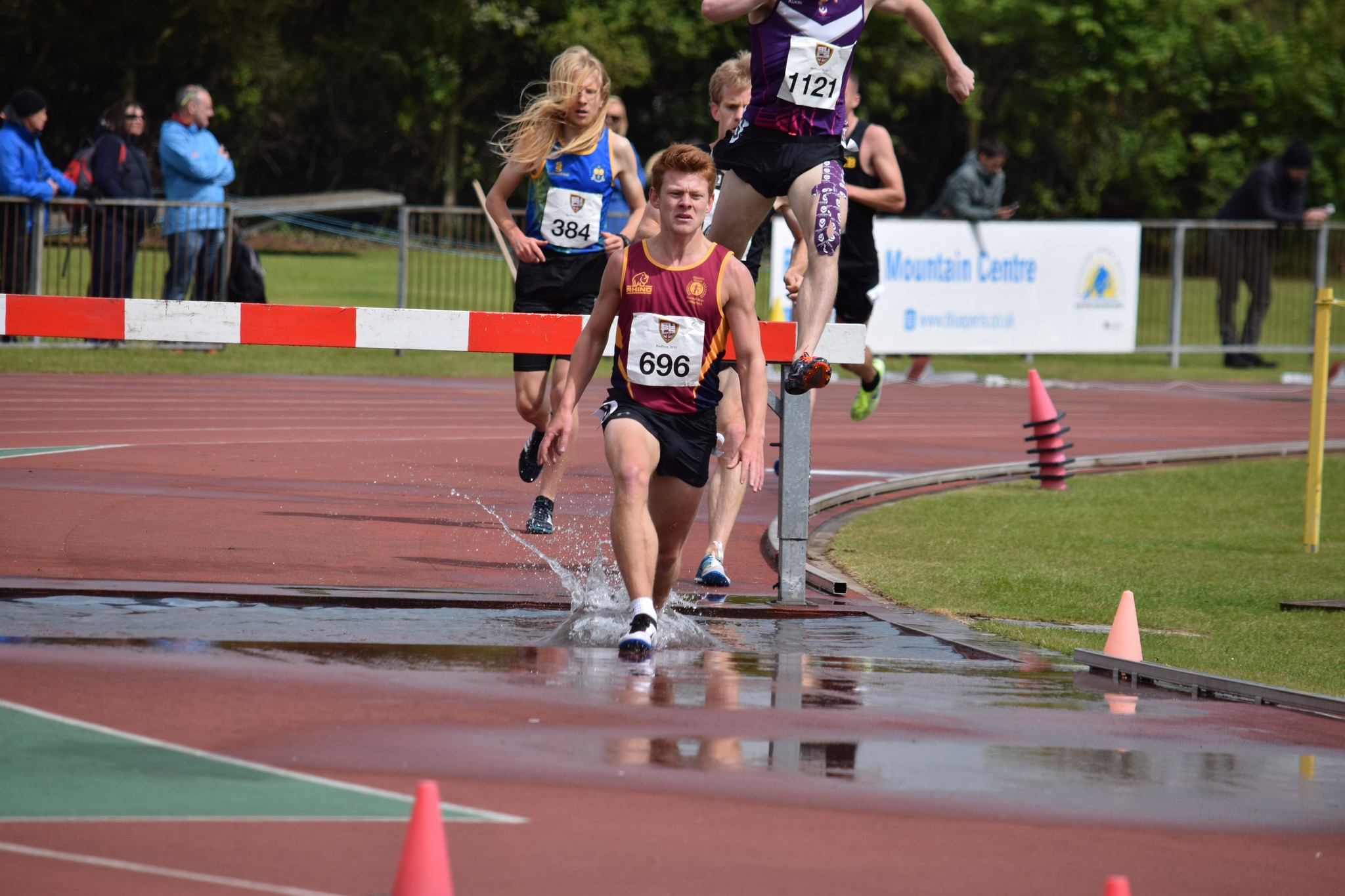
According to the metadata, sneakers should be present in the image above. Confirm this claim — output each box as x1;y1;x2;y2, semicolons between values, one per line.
694;541;731;585
526;496;555;534
784;345;833;394
849;358;887;422
698;594;728;602
617;654;653;676
519;411;554;482
617;613;658;650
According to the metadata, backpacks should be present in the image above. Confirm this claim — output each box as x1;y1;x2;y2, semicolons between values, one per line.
223;235;266;304
57;135;128;228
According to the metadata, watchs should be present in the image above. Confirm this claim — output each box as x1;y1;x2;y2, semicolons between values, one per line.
615;233;631;249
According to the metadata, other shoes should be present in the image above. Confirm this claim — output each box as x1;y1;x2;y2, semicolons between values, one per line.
1224;353;1275;367
906;357;929;382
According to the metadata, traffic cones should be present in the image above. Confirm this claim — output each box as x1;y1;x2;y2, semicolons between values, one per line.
1100;589;1145;662
1021;367;1076;489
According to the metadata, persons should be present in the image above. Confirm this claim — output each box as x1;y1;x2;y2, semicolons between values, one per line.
0;84;237;356
702;0;975;395
484;44;905;585
1211;141;1329;371
907;140;1017;386
536;146;765;663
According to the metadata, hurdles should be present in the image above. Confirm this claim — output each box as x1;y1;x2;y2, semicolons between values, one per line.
1;284;818;606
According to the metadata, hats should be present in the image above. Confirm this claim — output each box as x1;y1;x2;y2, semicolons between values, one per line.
1284;140;1313;169
12;90;46;118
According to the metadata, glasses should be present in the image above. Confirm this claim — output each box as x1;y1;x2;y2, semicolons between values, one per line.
124;113;148;121
